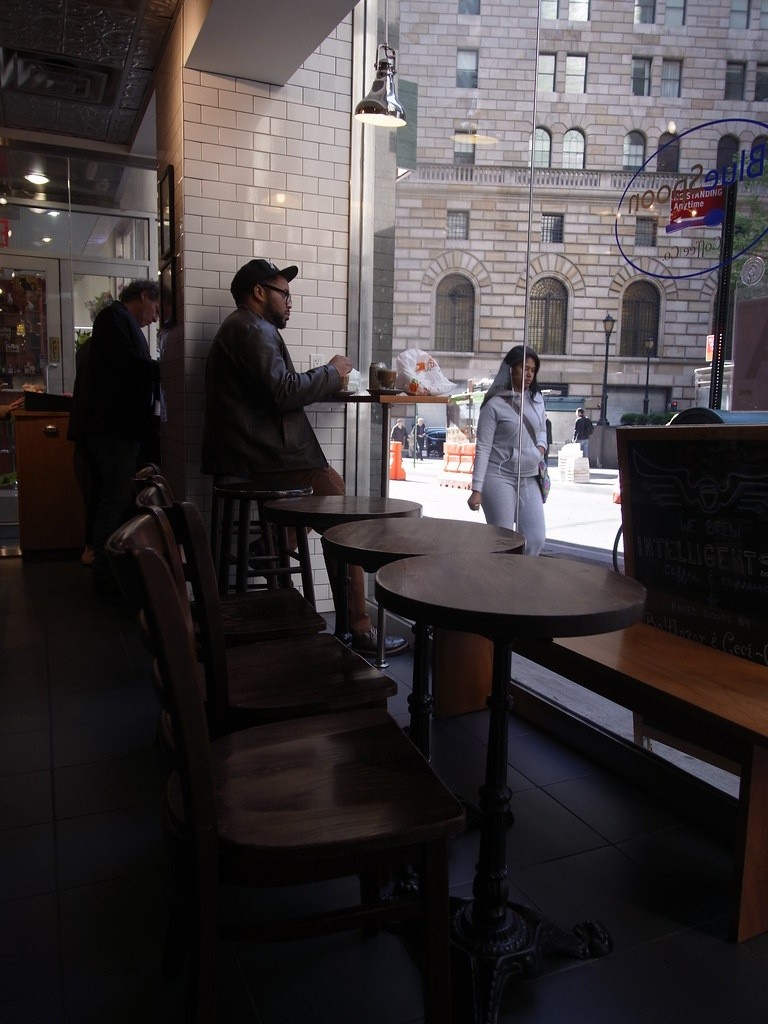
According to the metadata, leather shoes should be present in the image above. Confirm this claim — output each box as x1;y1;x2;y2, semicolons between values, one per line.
355;627;409;654
250;538;293;588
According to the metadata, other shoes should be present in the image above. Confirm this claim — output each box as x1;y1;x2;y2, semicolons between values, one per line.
81;548;96;564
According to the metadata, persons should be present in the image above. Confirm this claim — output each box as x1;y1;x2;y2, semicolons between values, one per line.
412;418;427;462
543;413;553;467
467;345;548;557
71;278;167;570
572;408;593;459
390;419;409;462
205;258;411;657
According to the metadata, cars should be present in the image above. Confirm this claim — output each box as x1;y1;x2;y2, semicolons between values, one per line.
424;427;448;457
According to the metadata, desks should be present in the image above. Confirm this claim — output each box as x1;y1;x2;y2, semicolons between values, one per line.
374;554;649;1024
264;495;421;650
322;517;526;903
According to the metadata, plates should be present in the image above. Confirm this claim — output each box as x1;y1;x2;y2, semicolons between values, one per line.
366;388;403;396
337;390;356;397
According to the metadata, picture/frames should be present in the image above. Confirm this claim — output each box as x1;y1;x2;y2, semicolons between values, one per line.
160;258;177;328
157;165;174;260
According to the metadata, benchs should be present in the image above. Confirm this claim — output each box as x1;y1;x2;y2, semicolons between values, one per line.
432;621;767;943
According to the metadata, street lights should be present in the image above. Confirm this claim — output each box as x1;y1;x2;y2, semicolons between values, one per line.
642;336;656;416
597;313;616;427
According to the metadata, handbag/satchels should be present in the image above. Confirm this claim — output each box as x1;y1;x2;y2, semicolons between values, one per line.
535;463;551;503
396;349;457;396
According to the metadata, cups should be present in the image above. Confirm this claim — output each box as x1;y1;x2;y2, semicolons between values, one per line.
375;369;396;389
340;374;349;391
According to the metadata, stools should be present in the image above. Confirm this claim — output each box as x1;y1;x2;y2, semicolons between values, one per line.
214;481;314;610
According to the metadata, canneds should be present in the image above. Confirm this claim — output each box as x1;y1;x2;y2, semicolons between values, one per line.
369;361;387;390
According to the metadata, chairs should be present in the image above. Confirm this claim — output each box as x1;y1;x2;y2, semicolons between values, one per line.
105;505;466;1024
135;475;399;740
131;462;326;646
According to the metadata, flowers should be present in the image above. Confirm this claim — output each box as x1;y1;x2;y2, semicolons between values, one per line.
73;292;113;352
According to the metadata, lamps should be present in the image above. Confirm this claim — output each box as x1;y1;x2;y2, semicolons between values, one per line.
353;0;407;127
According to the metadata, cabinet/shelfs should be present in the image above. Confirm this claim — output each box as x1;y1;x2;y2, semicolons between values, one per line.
11;405;87;564
0;274;45;475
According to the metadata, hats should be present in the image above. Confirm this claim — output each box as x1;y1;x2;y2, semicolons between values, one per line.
230;259;298;302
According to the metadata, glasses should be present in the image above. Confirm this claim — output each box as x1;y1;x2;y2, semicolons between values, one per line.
259;281;292;306
